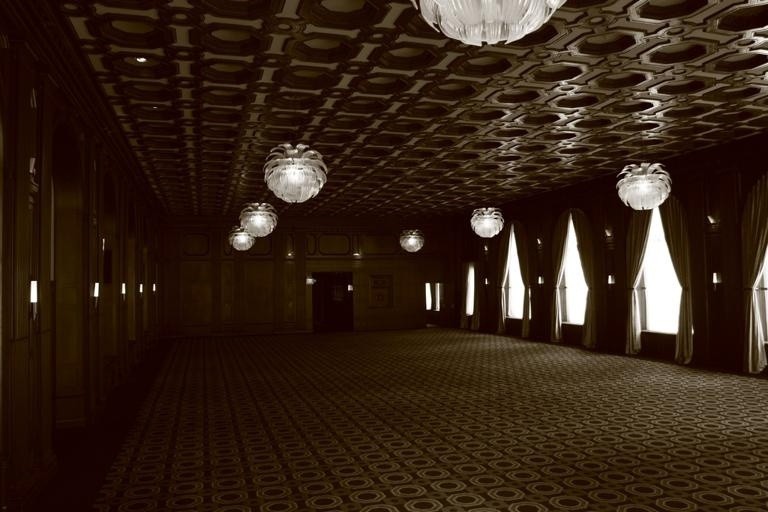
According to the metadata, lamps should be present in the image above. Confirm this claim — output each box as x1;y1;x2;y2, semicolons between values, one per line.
399;227;425;252
616;162;673;210
228;143;326;251
470;207;504;238
30;280;38;319
413;0;567;47
